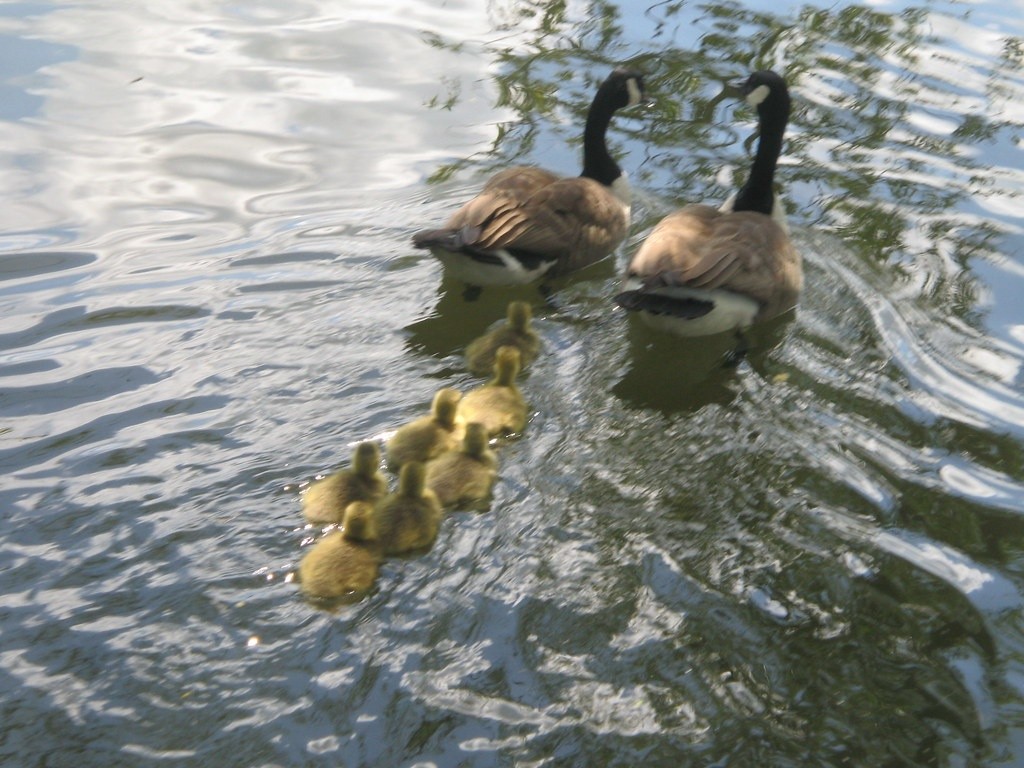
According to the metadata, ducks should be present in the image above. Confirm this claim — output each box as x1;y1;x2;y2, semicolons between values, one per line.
298;68;804;603
